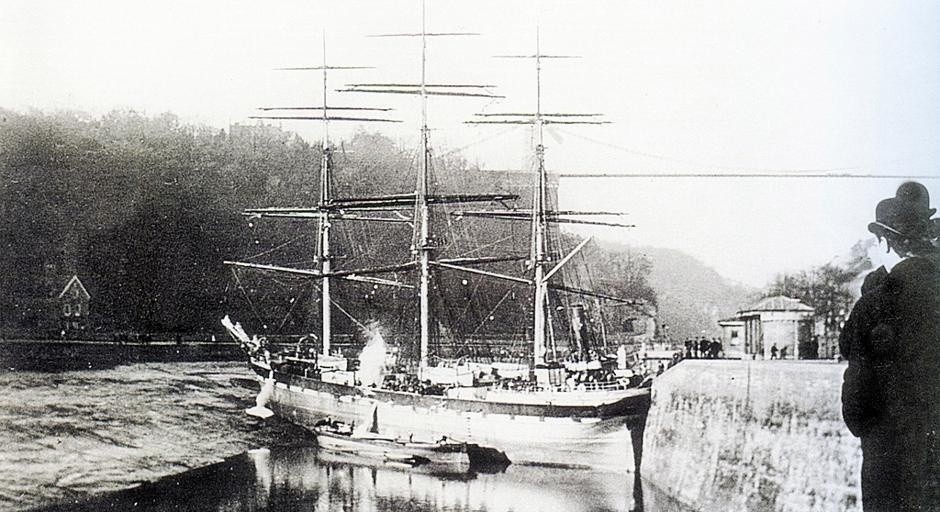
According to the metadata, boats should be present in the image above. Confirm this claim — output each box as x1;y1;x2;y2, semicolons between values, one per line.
315;427;469;479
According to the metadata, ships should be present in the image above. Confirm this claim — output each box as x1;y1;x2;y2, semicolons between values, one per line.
225;0;651;477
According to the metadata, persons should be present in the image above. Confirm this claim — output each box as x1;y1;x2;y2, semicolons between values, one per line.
839;180;938;509
266;337;787;402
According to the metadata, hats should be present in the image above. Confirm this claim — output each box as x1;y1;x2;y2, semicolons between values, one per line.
868;198;937;242
